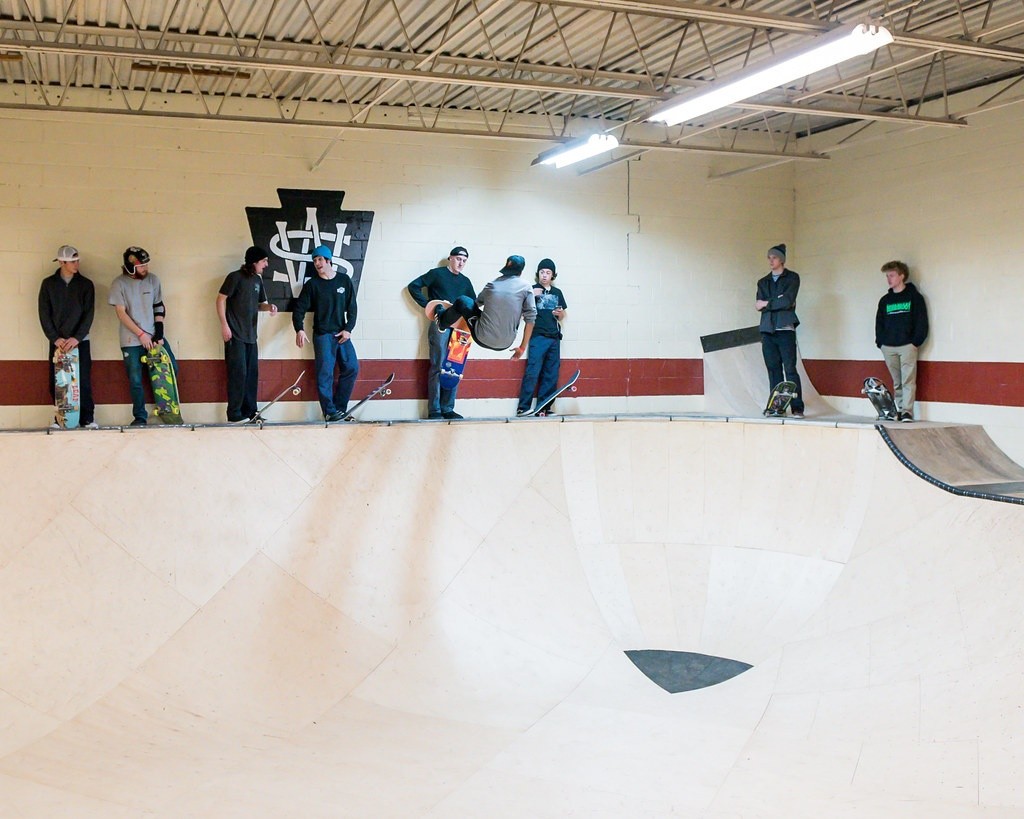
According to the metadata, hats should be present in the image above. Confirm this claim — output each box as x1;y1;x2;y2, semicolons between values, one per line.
312;245;333;260
244;245;268;264
767;244;786;261
447;247;468;260
499;255;526;276
52;244;79;262
537;258;555;274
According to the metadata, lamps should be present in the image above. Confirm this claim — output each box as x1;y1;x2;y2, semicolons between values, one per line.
529;130;620;170
632;16;895;128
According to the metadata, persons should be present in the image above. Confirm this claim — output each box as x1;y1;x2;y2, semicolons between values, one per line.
292;245;359;421
108;245;178;427
755;243;807;417
38;245;102;428
876;260;929;423
516;258;567;417
216;246;277;424
408;246;478;420
434;253;537;362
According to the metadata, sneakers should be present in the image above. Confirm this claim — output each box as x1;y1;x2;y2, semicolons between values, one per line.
325;412;342;422
517;408;535;417
337;409;356;421
433;304;447;333
535;409;558;416
227;415;251;424
248;414;265;423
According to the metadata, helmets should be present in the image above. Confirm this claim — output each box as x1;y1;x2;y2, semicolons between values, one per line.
123;246;150;275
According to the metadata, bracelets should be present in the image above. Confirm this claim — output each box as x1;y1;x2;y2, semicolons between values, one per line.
139;331;145;337
520;346;525;351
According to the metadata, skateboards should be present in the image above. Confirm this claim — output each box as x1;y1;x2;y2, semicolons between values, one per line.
140;343;185;425
247;369;305;425
860;376;899;422
324;371;395;424
424;299;471;333
52;344;81;429
523;369;582;417
763;380;798;418
439;328;473;390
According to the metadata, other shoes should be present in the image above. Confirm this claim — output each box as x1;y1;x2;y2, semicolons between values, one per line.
85;421;98;430
793;411;805;419
130;418;147;426
901;413;913;422
442;411;464;420
50;423;61;429
428;413;444;419
